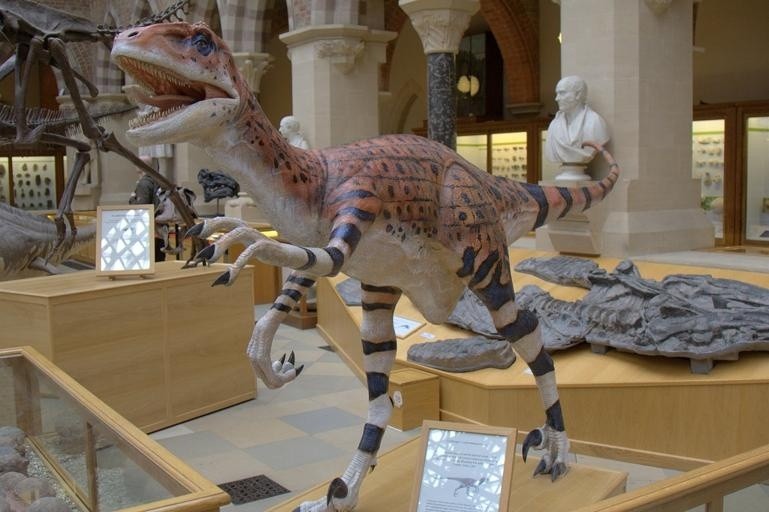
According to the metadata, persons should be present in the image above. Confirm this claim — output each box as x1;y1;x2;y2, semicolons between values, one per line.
543;74;611;162
127;154;175;261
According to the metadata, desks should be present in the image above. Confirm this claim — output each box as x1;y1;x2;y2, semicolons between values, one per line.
0;256;260;456
166;215;283;305
263;416;629;512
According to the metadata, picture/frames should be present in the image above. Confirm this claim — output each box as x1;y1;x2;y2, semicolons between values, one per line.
407;418;520;511
92;202;157;276
